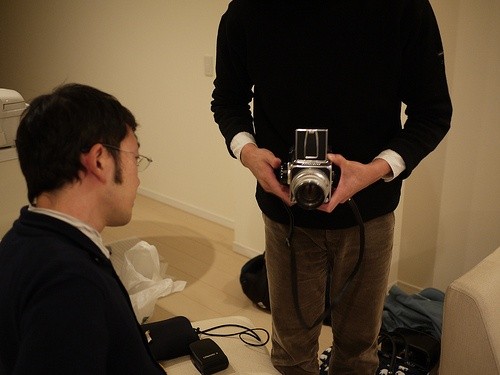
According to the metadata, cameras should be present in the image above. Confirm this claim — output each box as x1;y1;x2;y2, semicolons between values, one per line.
279;127;334;211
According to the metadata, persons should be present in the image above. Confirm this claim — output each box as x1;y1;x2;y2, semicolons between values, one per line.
0;82;167;374
210;0;452;375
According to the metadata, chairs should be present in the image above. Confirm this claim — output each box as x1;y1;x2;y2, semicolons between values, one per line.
436;247;500;375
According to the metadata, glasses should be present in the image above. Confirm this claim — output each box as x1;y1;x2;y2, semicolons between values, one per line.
87;143;154;173
